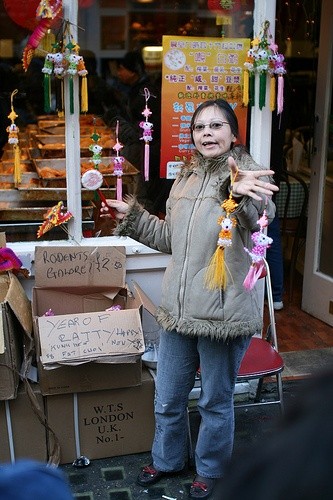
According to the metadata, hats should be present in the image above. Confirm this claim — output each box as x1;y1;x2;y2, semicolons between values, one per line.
121;51;146;75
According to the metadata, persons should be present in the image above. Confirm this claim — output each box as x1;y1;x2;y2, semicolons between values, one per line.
0;55;36;159
263;75;292;310
101;99;280;499
78;50;175;213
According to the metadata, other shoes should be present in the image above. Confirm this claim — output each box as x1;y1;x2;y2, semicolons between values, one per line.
138;463;165;487
190;474;218;499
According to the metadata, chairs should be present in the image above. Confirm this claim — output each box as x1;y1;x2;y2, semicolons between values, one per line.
277;171;310;306
186;257;283;459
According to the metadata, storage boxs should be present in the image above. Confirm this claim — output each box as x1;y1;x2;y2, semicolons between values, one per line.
0;232;156;464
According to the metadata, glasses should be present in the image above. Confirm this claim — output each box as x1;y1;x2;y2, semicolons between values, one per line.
189;121;229;131
